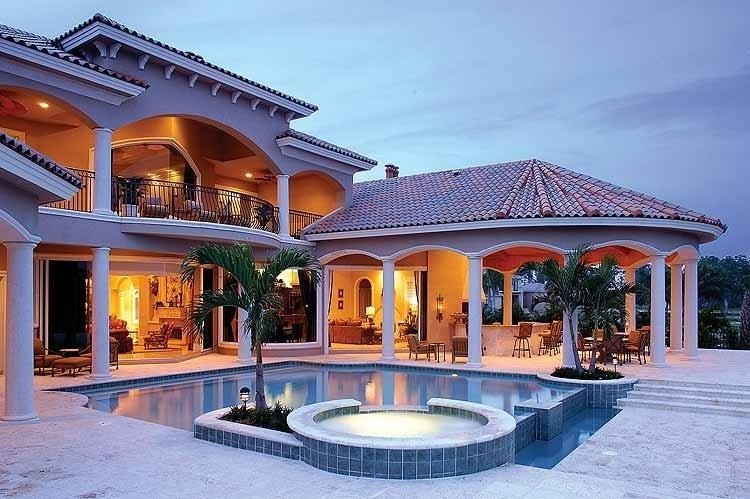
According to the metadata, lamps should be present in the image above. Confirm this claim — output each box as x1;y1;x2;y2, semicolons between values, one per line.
364;372;376;404
435;293;445;321
365;304;376;344
240;386;251;419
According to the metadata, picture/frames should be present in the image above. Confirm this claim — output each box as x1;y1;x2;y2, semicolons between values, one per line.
338;299;344;310
338;288;344;299
166;276;183;303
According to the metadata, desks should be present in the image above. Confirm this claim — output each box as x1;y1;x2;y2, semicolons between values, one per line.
427;342;446;363
60;348;82;374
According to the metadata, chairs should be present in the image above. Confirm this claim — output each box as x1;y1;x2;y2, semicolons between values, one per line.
576;324;651;363
405;334;437;362
275;319;305;344
34;338;65;376
450;335;468;363
50;336;120;378
109;318;176;354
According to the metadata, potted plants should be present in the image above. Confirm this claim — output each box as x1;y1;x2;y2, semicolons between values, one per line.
115;174;148;216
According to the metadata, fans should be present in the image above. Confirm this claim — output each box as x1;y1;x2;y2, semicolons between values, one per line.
254;170;277;184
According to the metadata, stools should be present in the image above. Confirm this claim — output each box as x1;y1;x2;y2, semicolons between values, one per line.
537;320;564;357
511;322;533;359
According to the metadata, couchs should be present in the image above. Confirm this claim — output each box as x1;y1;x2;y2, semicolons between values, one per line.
327;309;419;346
139;194;252;227
